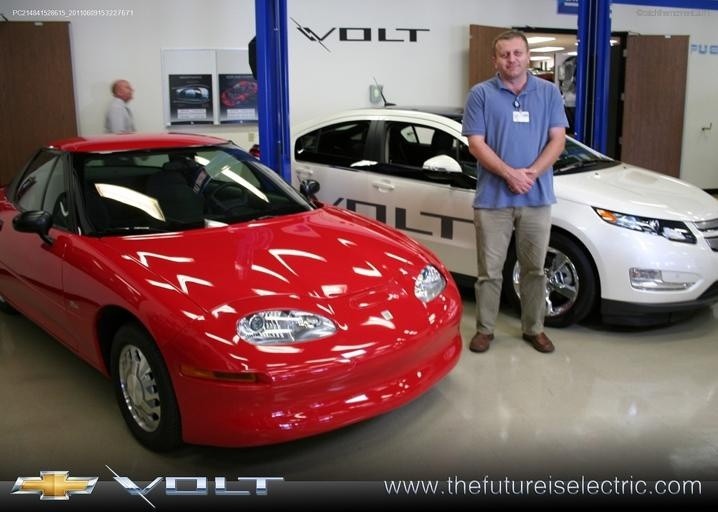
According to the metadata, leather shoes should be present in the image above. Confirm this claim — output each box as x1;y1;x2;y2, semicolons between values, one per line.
469;327;495;354
522;328;558;355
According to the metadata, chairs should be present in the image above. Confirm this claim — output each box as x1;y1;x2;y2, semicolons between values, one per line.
158;172;216;223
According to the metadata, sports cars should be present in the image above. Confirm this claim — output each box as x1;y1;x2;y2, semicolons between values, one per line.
170;85;211;104
221;81;258;107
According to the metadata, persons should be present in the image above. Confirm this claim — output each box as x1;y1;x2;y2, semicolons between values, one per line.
103;80;135;135
462;29;570;352
